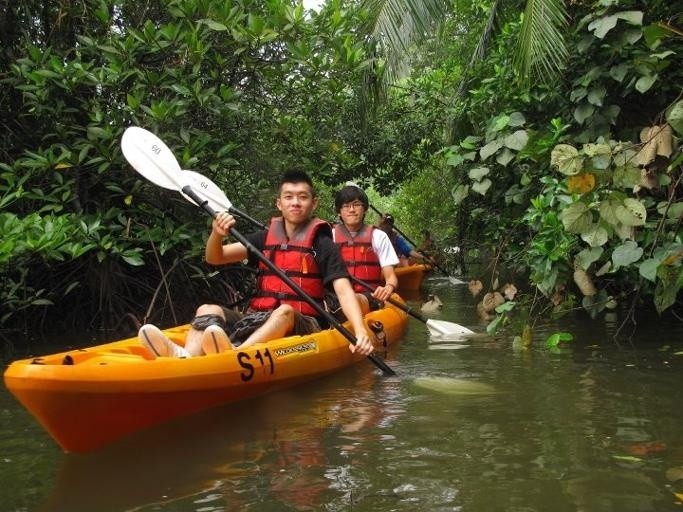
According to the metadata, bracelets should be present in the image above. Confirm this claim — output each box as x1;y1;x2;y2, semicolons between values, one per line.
385;283;396;290
422;256;425;262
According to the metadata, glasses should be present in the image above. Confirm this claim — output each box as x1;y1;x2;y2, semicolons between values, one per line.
341;202;364;211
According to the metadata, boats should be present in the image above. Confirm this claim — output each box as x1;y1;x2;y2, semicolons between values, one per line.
3;289;407;454
381;264;433;290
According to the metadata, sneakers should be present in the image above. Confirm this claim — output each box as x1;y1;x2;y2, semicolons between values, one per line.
138;324;193;361
201;325;236;357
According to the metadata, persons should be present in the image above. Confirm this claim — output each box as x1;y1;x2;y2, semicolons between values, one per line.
373;213;434;267
328;185;399;315
407;228;441;267
137;159;374;357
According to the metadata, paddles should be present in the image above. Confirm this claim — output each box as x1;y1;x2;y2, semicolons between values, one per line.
121;126;497;396
178;169;477;350
369;204;467;285
